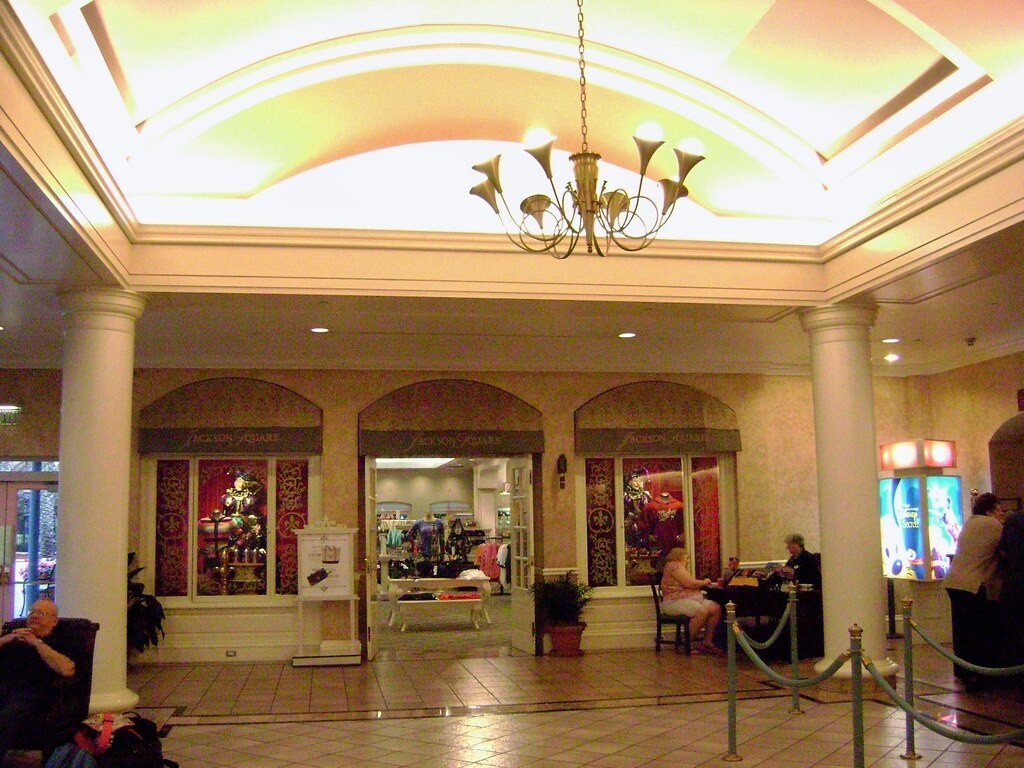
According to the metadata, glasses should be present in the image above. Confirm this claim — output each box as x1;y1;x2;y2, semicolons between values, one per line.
28;608;56;617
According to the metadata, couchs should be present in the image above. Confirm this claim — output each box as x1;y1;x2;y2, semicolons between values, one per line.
0;616;98;764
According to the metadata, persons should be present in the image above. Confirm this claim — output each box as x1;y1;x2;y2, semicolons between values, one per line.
445;517;472;563
197;472;267;594
406;512;446;558
624;473;685;584
943;492;1024;701
767;533;821;589
0;597;77;765
660;547;725;659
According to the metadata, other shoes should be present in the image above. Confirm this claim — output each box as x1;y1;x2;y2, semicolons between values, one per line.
690;641;724;659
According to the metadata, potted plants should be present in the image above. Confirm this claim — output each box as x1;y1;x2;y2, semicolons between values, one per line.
126;547;172;670
526;570;598;659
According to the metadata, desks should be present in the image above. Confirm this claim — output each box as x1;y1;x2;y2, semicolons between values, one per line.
698;583;821;661
385;576;492;631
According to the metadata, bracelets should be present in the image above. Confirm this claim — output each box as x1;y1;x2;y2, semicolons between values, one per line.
32;638;44;649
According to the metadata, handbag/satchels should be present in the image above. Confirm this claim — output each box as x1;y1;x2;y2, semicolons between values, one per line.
75;711;179;768
445;517;471;554
451;554;474;577
433;553;451;577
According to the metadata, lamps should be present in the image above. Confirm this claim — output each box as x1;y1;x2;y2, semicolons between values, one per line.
468;0;707;260
558;453;568;490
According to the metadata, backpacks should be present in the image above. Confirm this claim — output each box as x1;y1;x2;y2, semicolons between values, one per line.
43;744;98;768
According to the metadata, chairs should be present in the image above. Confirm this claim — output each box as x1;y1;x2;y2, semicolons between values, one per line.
648;570;690;650
